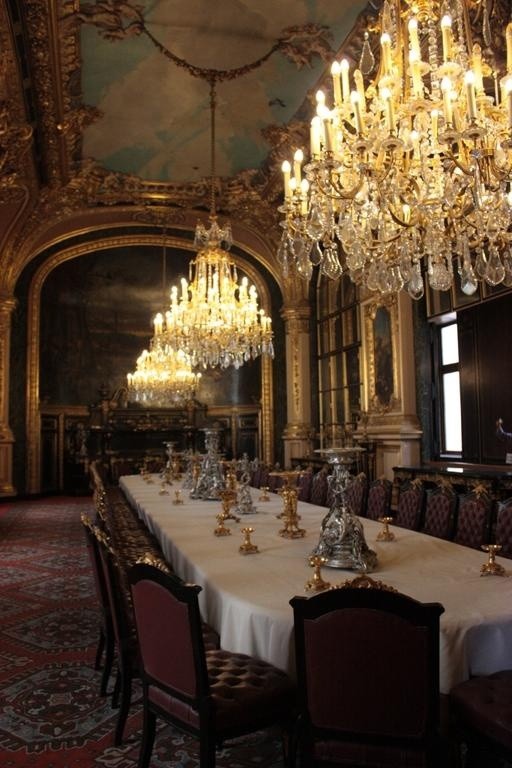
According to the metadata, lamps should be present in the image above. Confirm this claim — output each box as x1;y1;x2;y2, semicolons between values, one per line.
277;0;512;301
81;1;337;369
98;179;217;409
